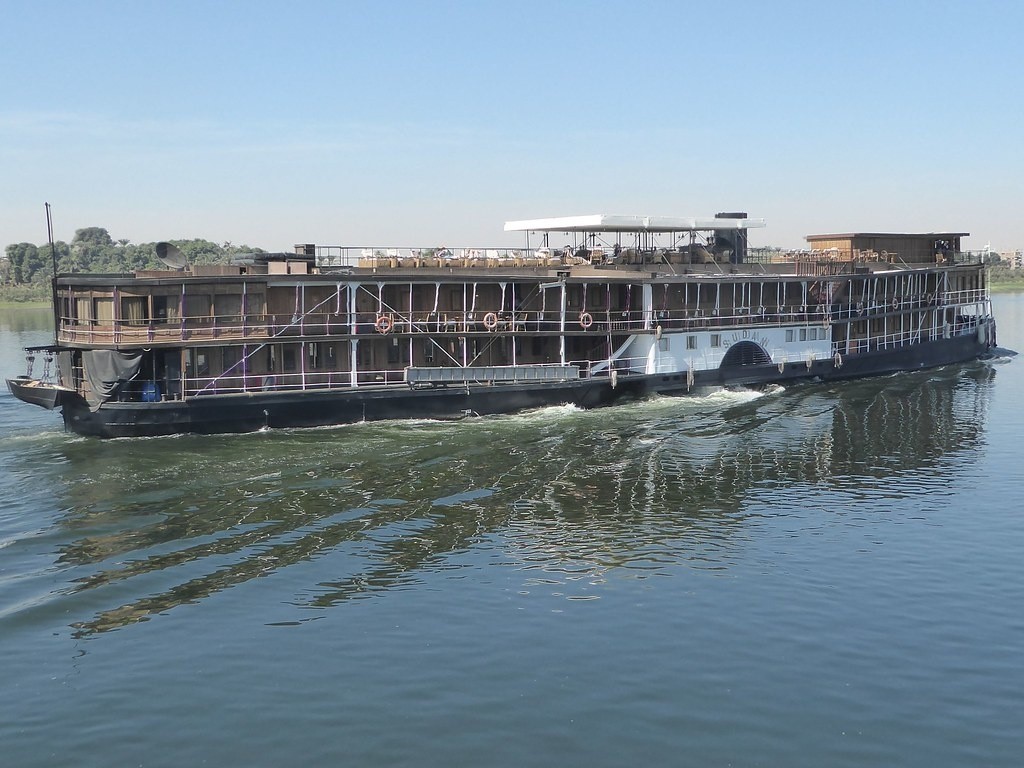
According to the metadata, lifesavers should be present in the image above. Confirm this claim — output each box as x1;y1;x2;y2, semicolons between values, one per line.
376;317;391;332
892;298;899;310
927;294;933;304
857;304;864;315
580;312;593;328
483;313;497;329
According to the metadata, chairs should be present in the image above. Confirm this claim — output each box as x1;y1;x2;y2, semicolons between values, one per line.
592;250;602;265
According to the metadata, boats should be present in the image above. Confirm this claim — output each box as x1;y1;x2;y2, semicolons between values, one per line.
5;197;1001;436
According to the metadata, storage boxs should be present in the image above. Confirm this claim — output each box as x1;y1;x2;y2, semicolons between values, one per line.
358;256;561;268
628;248;731;264
